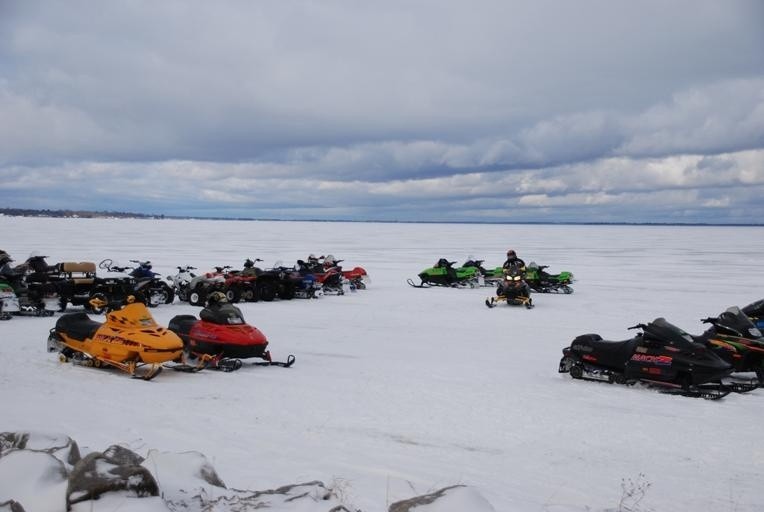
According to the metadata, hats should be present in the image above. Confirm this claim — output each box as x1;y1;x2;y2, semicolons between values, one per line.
215;292;227;303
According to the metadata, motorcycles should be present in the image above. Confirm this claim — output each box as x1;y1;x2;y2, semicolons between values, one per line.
558;298;764;401
403;255;578;308
164;299;296;374
0;251;174;322
169;252;366;306
44;295;184;381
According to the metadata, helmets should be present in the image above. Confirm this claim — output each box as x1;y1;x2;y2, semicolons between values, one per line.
507;250;516;259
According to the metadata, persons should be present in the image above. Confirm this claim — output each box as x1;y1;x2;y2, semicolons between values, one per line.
199;292;243;325
495;249;531;299
